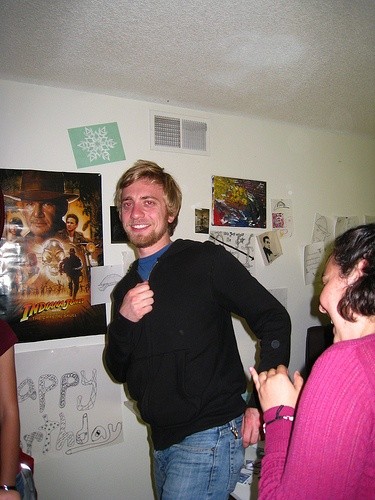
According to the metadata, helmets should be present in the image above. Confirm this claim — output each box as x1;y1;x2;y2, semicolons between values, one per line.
8;217;24;234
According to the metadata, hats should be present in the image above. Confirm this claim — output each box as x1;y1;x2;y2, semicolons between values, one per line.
66;248;77;254
10;170;80;204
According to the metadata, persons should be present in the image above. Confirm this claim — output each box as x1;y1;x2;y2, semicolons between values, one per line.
250;223;374;500
102;158;295;500
1;313;24;500
0;169;95;301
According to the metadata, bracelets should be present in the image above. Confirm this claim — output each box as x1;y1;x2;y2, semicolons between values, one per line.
1;485;18;492
